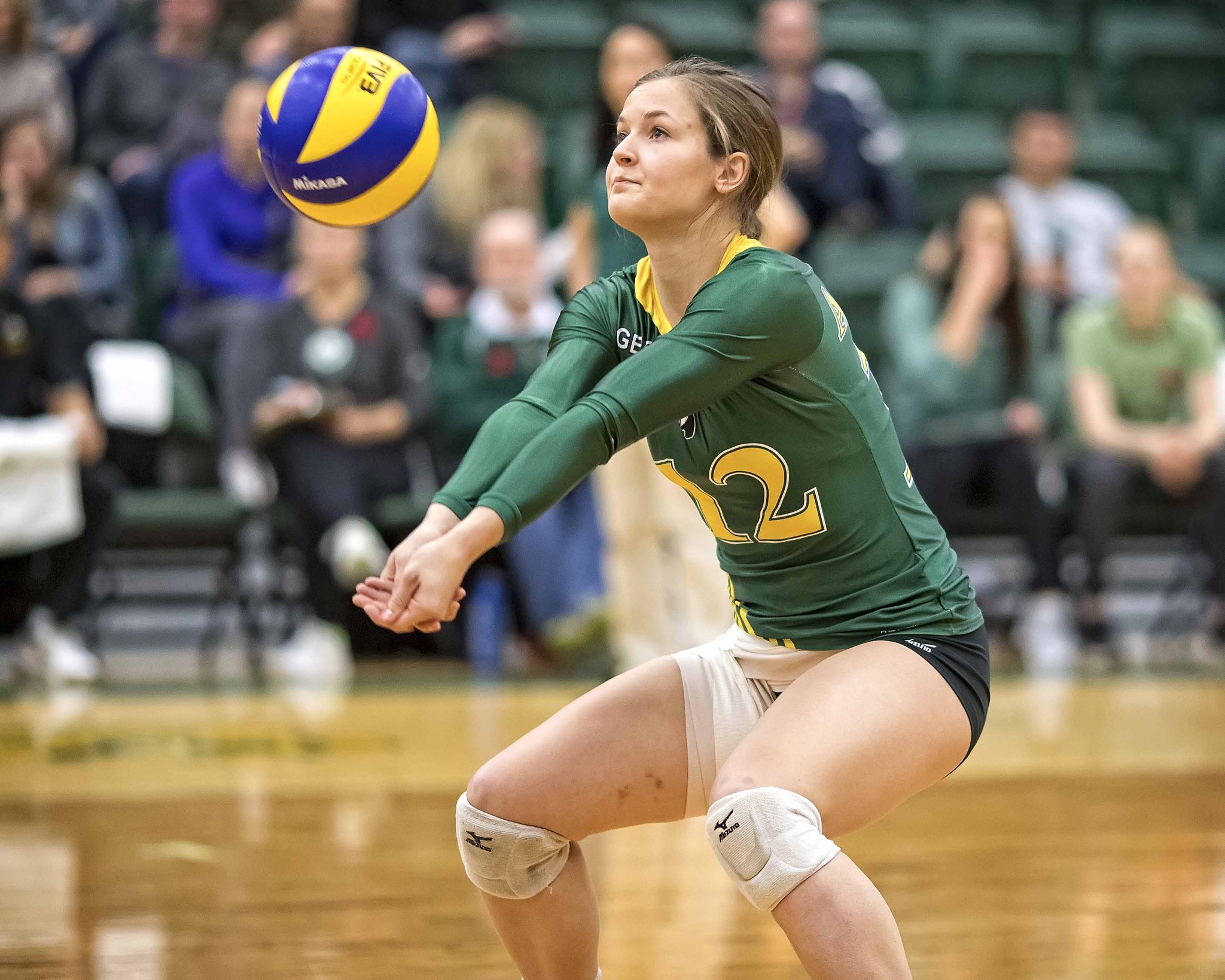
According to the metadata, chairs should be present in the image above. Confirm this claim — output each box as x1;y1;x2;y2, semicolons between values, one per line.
0;0;1225;690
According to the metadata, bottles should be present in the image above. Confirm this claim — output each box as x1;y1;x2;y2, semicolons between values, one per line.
464;576;501;662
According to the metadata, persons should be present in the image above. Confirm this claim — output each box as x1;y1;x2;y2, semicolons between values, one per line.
352;54;994;980
0;0;1225;733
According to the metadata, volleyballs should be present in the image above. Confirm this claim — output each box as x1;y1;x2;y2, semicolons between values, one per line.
256;46;441;229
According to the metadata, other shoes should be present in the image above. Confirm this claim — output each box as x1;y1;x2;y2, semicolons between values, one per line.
264;622;351;685
14;601;104;683
1011;587;1081;678
219;445;272;505
325;513;385;587
